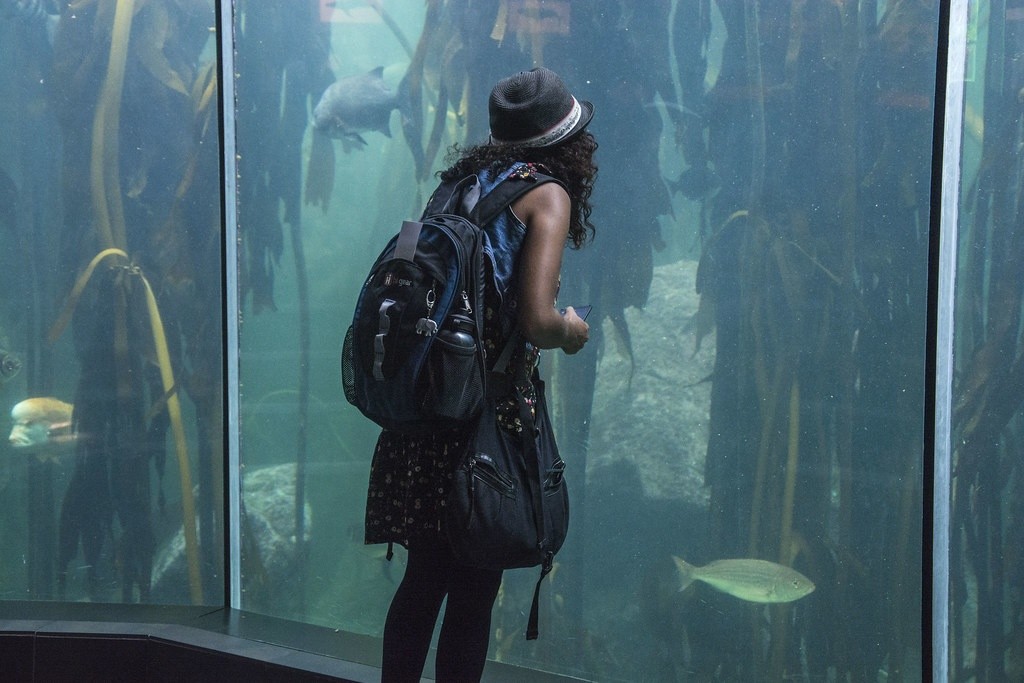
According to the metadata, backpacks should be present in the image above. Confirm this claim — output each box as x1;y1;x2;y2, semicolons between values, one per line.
342;168;570;435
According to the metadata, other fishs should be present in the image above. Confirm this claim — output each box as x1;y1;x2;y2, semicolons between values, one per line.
311;64;414;146
672;555;815;603
9;398;80;455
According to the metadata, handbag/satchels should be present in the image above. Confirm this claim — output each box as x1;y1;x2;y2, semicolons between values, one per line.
440;379;568;569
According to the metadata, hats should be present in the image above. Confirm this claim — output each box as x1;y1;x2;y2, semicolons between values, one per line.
489;67;595;149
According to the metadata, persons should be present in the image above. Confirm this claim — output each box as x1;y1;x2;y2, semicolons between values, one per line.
365;66;599;683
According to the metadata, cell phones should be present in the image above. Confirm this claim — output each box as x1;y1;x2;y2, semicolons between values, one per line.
559;305;593;322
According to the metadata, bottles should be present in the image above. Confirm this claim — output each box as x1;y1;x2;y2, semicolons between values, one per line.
438;315;477;348
